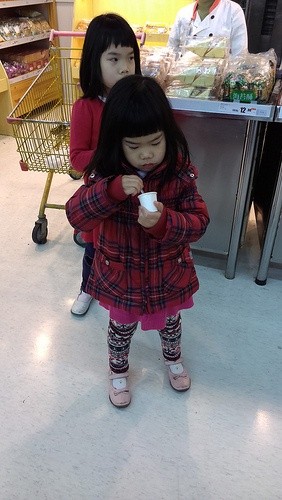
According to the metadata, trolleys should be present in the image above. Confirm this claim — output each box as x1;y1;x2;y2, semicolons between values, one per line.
7;28;146;247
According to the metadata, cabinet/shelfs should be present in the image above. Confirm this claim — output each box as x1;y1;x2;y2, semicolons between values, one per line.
0;0;64;118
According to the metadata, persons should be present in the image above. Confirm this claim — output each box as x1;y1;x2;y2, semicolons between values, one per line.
65;75;212;409
166;0;249;56
70;12;143;316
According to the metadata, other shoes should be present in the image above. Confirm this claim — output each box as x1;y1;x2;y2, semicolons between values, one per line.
108;369;131;407
71;290;94;315
165;358;190;392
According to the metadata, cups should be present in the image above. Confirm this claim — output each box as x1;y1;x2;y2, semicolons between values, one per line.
137;192;158;213
